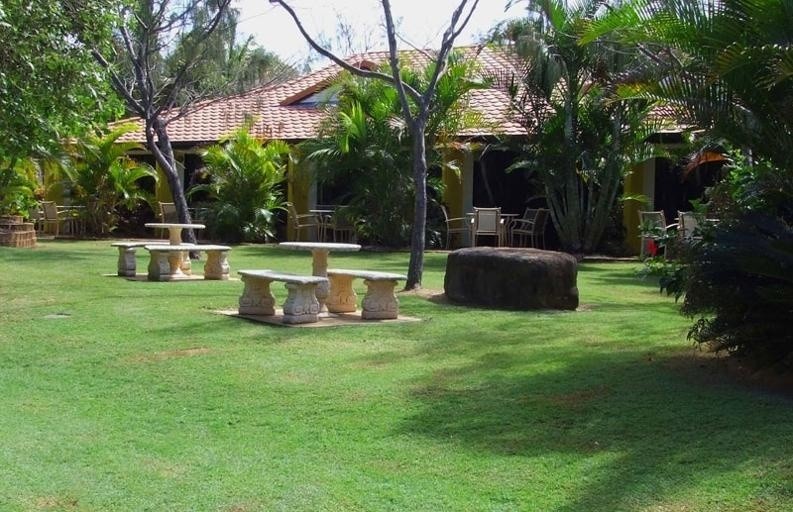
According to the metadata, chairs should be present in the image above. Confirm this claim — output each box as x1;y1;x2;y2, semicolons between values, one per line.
158;201;185;239
636;209;708;262
287;202;359;245
440;203;551;251
32;199;87;237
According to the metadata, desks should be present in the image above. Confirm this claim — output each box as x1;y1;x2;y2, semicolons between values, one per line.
110;240;232;282
278;241;363;315
144;222;207;278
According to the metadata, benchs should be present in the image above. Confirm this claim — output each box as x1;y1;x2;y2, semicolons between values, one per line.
237;268;408;324
